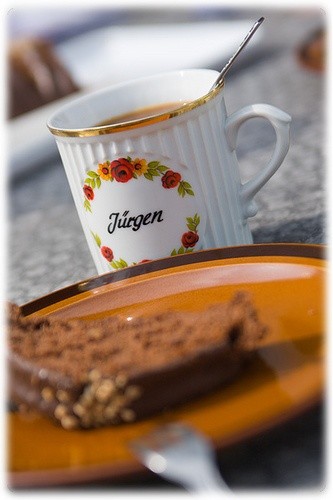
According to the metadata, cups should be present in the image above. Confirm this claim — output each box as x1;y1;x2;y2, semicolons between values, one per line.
46;69;293;275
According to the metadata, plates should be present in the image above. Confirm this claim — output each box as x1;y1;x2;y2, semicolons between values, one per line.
6;243;327;490
8;21;260;183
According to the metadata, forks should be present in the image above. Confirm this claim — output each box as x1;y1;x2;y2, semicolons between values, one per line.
123;422;235;492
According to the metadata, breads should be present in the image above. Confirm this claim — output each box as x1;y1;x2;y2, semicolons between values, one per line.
7;290;270;429
